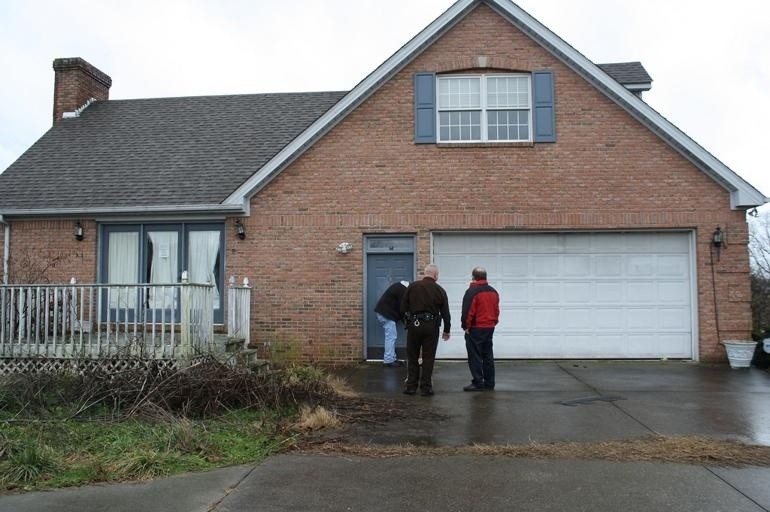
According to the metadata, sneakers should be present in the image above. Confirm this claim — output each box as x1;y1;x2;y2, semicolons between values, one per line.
420;388;435;397
383;361;403;368
402;388;416;395
463;381;494;393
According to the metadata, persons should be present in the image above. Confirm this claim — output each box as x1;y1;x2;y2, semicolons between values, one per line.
460;266;501;392
399;262;451;396
373;279;414;368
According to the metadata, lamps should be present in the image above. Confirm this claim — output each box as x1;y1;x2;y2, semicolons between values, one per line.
713;224;723;247
235;218;246;240
75;222;83;241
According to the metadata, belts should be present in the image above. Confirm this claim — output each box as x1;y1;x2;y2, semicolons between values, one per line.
417;312;442;321
